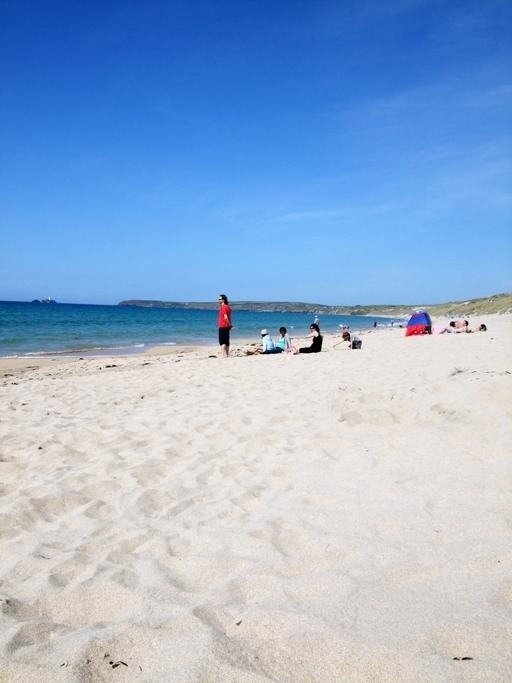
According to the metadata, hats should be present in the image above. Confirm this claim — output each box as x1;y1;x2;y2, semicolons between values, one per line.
261;329;267;334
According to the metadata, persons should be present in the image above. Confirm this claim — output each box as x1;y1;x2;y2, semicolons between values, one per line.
439;319;469;333
373;320;377;328
313;314;320;329
390;317;394;326
242;324;362;355
217;293;235;360
467;322;486;333
448;320;463;328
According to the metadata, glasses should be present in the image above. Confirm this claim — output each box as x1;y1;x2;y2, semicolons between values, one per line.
310;328;315;329
219;299;221;300
